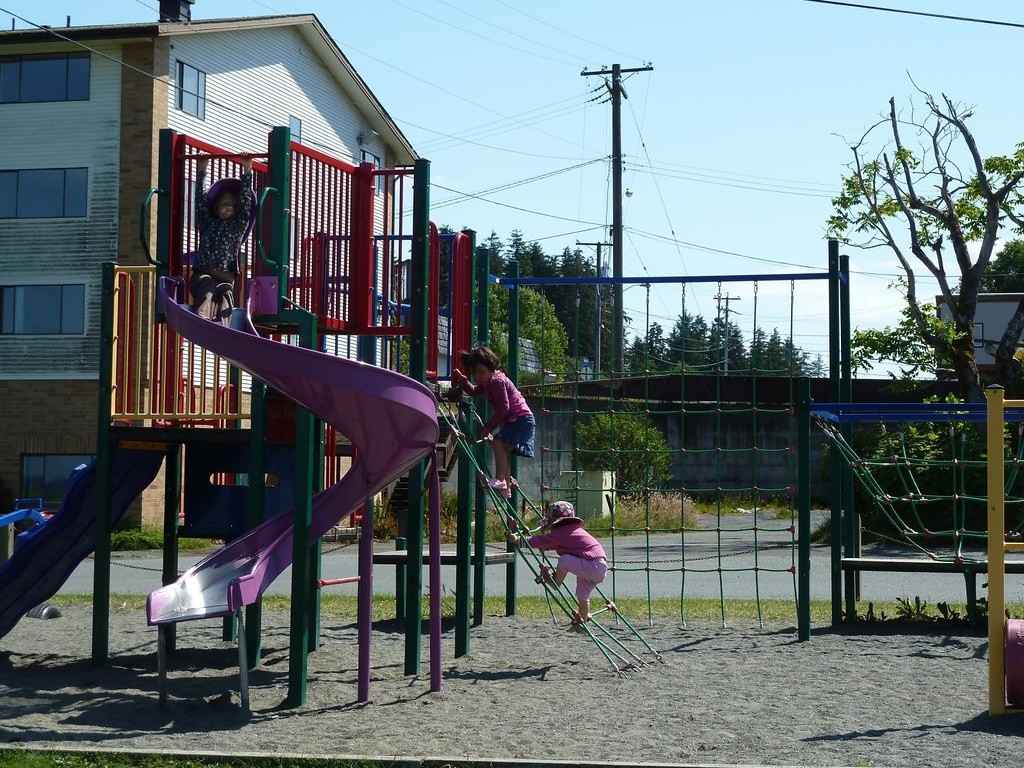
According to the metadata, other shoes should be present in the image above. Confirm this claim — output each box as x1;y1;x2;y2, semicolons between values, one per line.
482;484;511;498
486;477;507;489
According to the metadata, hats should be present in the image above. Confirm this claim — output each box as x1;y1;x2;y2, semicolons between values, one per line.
538;500;584;528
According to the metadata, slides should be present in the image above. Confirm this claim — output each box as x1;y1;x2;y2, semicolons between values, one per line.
134;275;443;628
1;443;167;643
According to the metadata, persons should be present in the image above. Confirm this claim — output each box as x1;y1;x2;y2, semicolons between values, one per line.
509;500;607;633
189;151;253;327
452;346;535;498
